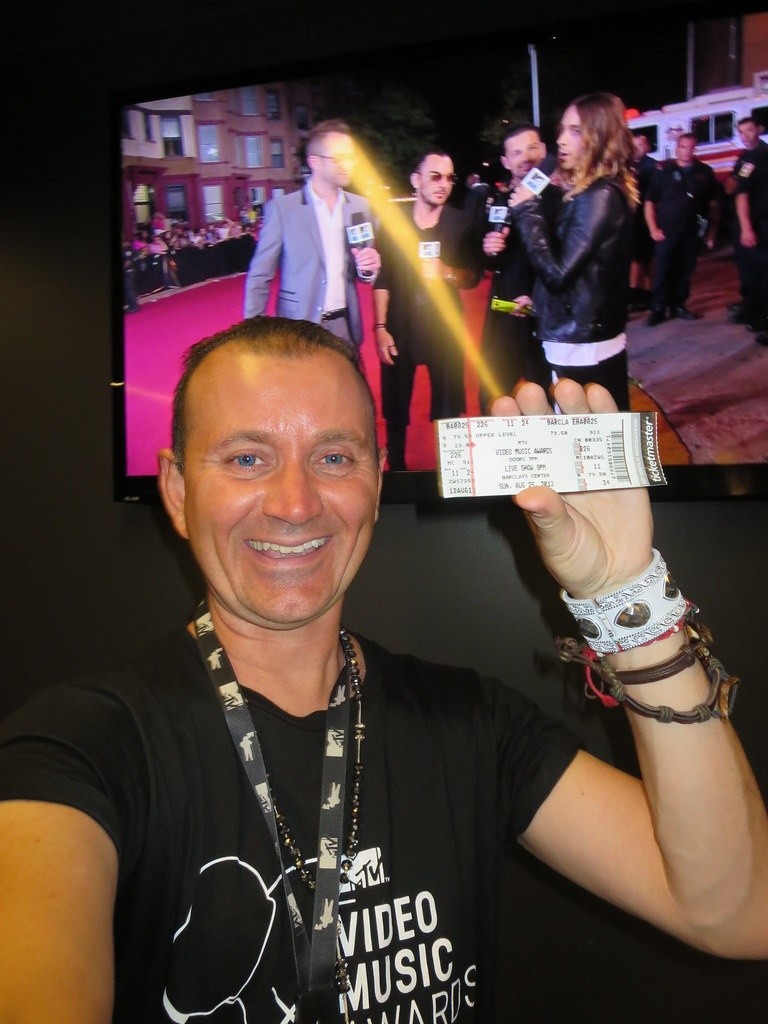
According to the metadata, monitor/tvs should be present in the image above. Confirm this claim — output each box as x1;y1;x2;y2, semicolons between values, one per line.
105;0;768;502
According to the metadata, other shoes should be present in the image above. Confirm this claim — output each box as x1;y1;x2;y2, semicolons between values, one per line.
643;306;666;327
671;304;697;320
728;298;768;346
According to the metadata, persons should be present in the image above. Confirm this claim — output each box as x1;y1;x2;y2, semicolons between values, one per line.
0;317;768;1024
125;89;768;469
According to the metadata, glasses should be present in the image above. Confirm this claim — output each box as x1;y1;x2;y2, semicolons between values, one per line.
418;170;459;183
318;155;356;166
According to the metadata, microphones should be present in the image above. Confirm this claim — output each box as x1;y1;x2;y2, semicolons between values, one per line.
419;228;441;259
346;212;375;277
521;154;558;196
488;193;513;256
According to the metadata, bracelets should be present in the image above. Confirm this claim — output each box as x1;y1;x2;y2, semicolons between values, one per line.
556;547;740;725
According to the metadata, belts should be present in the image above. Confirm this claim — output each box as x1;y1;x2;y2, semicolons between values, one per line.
322;308;347;321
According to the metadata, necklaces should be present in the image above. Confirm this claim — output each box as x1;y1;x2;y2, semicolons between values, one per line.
206;629;367;992
